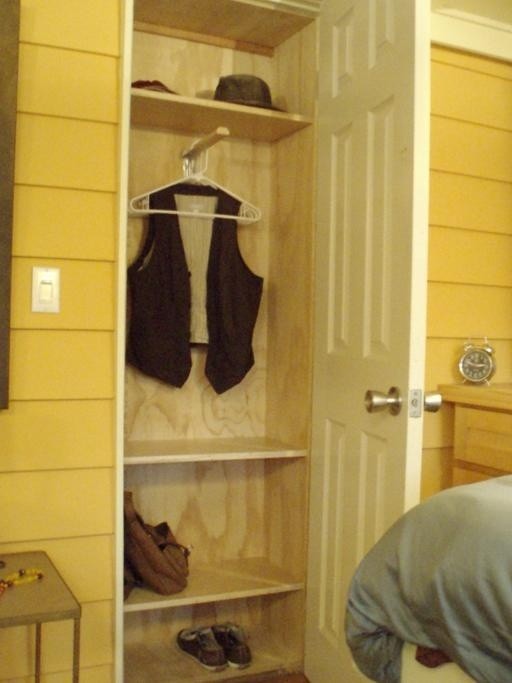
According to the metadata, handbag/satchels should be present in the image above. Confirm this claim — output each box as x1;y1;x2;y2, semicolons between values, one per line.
124;492;190;594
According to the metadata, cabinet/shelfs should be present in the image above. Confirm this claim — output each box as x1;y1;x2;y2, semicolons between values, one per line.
110;0;333;680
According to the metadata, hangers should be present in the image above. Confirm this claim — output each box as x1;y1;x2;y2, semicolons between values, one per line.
128;139;265;226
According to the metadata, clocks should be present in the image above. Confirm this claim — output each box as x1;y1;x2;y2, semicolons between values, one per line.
455;337;497;388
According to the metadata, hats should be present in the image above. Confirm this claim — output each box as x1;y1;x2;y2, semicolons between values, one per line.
213;74;287;111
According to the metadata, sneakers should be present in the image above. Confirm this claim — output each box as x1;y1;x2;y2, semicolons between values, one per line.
175;623;252;672
